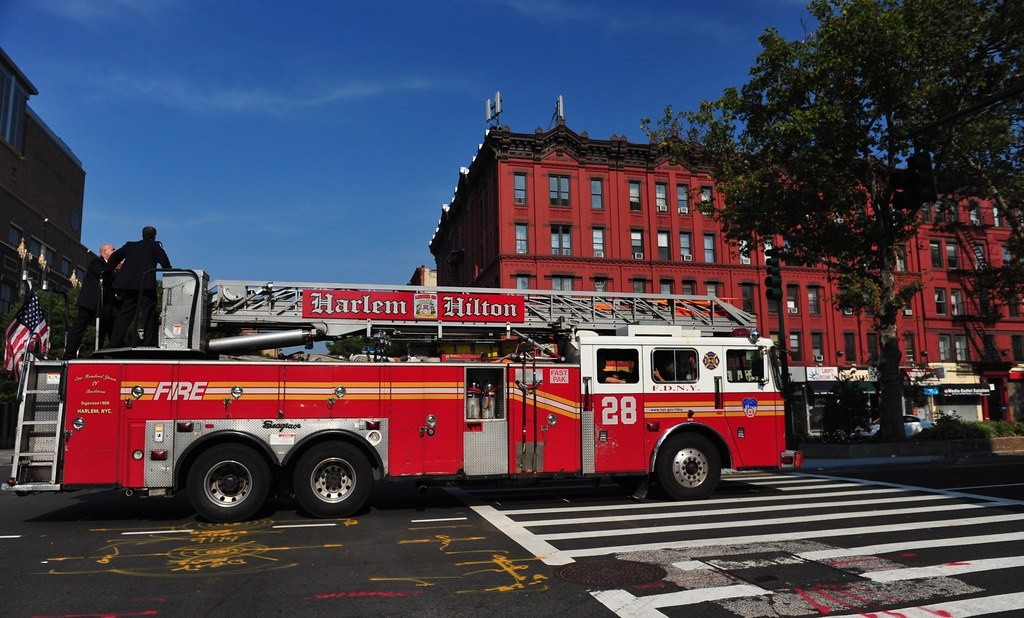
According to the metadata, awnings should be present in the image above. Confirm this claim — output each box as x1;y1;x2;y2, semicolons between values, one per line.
939;384;991;396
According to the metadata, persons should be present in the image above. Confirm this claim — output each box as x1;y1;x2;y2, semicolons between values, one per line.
62;226;172;360
597;352;674;383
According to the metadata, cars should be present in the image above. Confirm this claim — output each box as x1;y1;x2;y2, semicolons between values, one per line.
856;414;934;440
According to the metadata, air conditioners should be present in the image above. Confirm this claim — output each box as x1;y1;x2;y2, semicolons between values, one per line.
790;308;797;314
815;355;823;362
681;207;689;214
634;252;644;260
684;254;693;262
596;252;604;258
971;219;980;225
845;308;853;315
660;205;668;212
743;258;751;264
904;309;912;316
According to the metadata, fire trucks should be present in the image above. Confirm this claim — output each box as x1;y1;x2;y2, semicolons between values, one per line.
0;265;802;523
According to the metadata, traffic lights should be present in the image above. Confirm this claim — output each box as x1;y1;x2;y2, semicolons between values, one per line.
765;248;783;303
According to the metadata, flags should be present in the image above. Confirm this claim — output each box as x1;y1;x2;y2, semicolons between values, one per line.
0;294;51;383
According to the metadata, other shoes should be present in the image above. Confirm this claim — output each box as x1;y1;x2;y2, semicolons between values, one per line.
62;351;77;360
